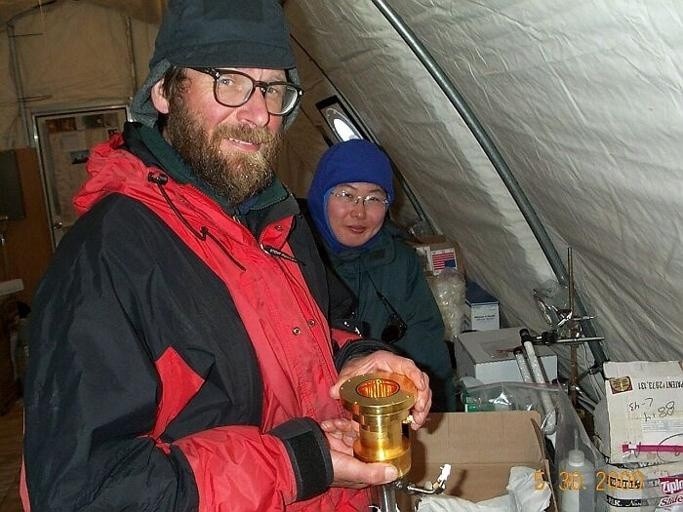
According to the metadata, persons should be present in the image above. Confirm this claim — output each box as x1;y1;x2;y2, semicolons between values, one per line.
20;0;434;512
301;139;458;413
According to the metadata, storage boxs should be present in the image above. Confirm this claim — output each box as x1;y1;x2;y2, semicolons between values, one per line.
371;410;558;512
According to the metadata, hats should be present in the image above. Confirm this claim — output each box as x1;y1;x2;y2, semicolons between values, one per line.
130;1;301;133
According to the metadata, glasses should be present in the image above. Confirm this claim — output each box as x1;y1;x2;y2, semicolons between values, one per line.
353;293;407;343
188;66;303;116
331;191;389;207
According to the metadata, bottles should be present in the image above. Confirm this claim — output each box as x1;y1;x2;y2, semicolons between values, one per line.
556;449;596;512
